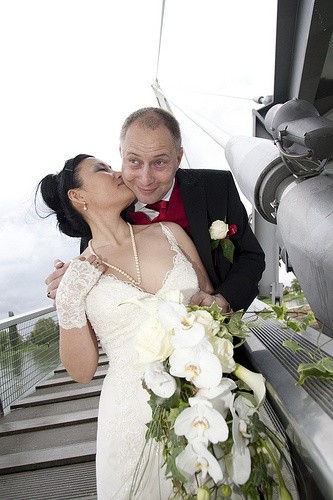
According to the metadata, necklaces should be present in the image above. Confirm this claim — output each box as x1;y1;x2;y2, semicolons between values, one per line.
88;222;142;286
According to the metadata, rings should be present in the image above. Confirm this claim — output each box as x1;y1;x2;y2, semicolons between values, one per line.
47;291;51;298
94;261;99;267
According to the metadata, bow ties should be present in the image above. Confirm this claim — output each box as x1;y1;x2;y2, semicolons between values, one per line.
144;200;169;214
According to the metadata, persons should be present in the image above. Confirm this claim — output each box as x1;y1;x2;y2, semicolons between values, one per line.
35;153;299;500
44;105;269;499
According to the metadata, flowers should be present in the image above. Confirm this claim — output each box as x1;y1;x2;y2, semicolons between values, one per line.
208;214;237;265
129;297;333;500
